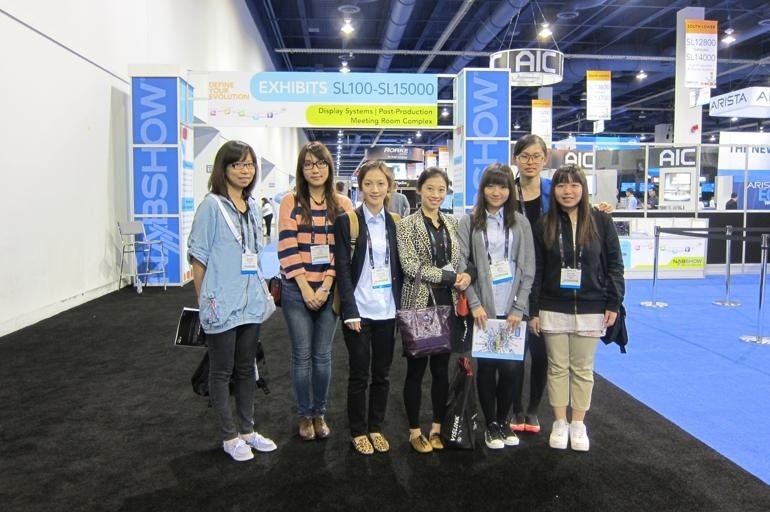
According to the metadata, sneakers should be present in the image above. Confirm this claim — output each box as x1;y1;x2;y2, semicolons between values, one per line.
223;432;255;462
485;411;590;455
237;428;278;453
298;415;444;455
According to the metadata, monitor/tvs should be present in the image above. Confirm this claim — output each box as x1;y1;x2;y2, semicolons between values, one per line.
648;168;659;179
639;181;654;192
701;183;715;194
620;180;637;192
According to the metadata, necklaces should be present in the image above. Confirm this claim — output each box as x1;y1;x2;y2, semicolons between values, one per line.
310;194;326;205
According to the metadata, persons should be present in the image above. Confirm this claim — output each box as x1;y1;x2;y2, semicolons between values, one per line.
333;180;346;196
508;135;613;431
395;168;478;452
458;163;536;449
624;186;636;210
529;165;625;452
388;182;410;220
189;140;277;461
261;197;273;237
645;188;658;209
334;161;404;455
726;192;738;210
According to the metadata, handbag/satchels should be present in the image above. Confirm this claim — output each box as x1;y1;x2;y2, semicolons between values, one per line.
269;276;282;307
600;304;628;352
454;292;473;353
192;337;270;405
441;357;485;449
396;305;453;359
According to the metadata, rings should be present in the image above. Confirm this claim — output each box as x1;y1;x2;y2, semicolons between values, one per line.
321;303;324;304
464;280;467;282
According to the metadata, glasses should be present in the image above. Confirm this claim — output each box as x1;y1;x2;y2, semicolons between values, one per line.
515;152;546;165
301;160;328;170
227;162;256;170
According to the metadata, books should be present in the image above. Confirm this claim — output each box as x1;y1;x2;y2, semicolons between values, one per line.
173;306;209;349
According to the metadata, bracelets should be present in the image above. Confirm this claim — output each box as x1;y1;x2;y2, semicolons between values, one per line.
320;286;330;295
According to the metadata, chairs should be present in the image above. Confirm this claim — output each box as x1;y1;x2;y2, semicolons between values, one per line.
117;221;167;293
